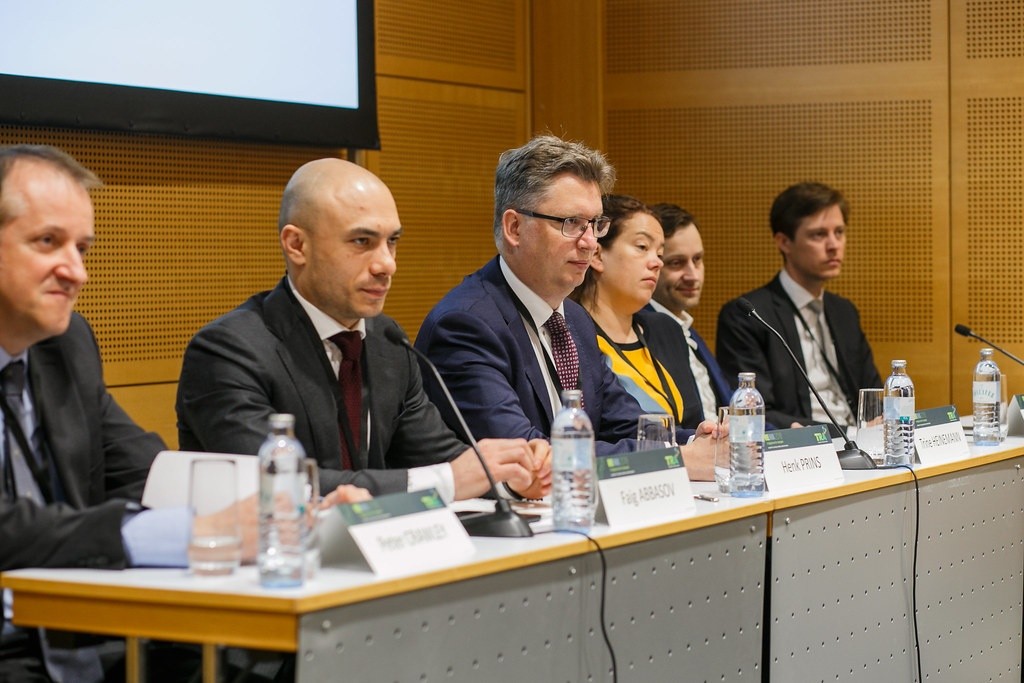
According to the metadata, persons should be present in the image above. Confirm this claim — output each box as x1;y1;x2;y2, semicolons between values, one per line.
413;136;730;483
0;143;374;683
569;194;683;427
175;158;553;502
647;203;778;431
716;183;884;438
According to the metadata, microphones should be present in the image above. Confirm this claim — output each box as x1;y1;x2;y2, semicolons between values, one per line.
736;295;877;469
954;324;1024;365
383;325;533;539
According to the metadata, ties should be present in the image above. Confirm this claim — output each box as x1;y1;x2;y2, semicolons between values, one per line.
807;298;855;427
0;359;104;683
324;331;363;470
543;312;584;413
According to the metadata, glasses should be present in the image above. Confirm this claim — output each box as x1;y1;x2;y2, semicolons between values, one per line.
499;205;613;238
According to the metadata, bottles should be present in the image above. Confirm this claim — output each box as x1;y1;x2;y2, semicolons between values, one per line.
973;348;1000;446
549;388;595;535
255;413;308;590
729;372;766;497
882;359;915;468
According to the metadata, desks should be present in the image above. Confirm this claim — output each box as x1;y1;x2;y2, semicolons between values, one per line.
0;421;1024;682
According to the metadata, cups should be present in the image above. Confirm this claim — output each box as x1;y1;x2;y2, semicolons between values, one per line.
855;388;885;467
714;406;731;493
636;415;677;452
187;459;242;577
300;458;322;578
998;375;1008;442
594;480;600;510
